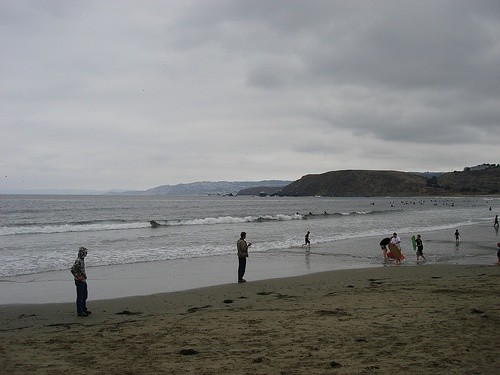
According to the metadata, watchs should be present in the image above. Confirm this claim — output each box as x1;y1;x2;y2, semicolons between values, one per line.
71;247;93;316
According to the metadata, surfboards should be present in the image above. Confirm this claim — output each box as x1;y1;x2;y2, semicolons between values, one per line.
387;243;401;263
388;252;405;260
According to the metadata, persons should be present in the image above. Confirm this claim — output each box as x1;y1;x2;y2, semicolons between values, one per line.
493;214;499;226
302;230;311;248
489;206;492;211
380;232;402;264
415;235;423;263
296;211;327;215
236;232;252;282
496;242;500;264
454;228;461;242
370;197;455;209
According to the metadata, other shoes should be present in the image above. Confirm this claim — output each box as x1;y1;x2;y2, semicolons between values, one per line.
78;312;88;317
85;311;92;315
238;279;246;283
